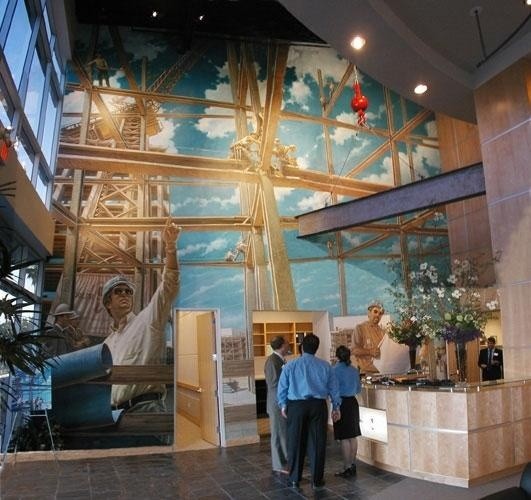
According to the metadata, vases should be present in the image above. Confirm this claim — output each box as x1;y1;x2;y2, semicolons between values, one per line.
455;344;468;384
409;343;416;370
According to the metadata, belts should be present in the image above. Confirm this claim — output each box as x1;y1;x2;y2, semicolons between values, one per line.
112;392;162;410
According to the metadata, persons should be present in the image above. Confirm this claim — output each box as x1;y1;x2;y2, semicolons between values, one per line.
332;345;362;476
44;303;75;357
101;215;181;427
477;337;502;381
277;332;342;490
350;301;392;374
86;52;112;87
66;310;92;350
264;335;289;473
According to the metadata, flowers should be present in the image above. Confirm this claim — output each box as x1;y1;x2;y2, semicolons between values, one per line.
385;307;427;344
405;251;507;343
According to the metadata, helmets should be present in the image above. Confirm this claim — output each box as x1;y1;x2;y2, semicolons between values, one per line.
52;304;73;316
102;276;136;304
70;310;80;319
368;301;384;312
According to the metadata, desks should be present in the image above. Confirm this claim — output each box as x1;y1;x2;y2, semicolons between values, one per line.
255;375;269;417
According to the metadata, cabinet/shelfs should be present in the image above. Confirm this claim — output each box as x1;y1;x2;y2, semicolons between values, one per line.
253;322;313;360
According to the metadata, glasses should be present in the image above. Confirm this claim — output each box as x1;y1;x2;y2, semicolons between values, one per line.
108;287;134;296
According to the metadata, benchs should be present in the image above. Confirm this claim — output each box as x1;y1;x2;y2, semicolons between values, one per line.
478;462;531;500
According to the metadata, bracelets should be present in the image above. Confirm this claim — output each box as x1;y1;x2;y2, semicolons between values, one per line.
163;247;177;254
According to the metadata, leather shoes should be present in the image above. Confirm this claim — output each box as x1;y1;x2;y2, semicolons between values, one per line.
313;480;325;489
291;480;299;488
282;466;289;473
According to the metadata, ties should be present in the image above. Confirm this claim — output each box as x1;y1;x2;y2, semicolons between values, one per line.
489;350;492;363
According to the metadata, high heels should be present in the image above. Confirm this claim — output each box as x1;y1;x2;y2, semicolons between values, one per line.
335;463;356;476
